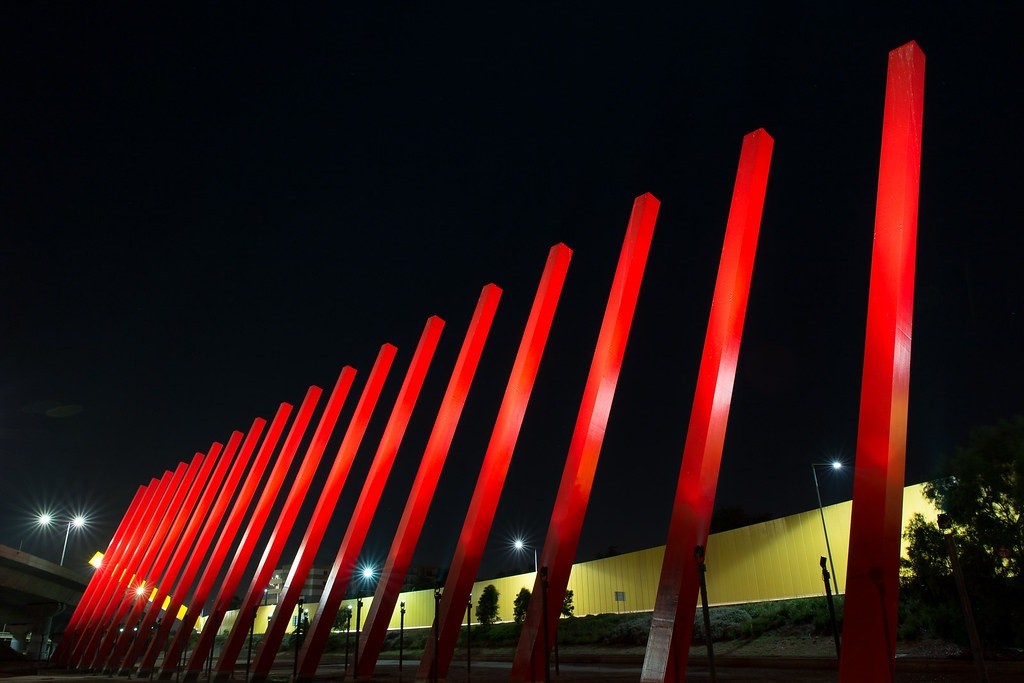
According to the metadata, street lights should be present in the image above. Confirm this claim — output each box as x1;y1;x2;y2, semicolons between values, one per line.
39;516;88;566
514;539;538;574
810;461;842;597
